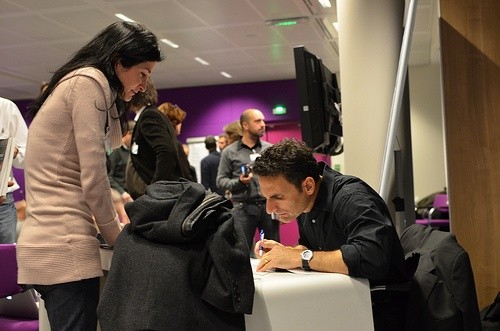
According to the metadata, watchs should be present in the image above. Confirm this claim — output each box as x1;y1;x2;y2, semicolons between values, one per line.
301;249;313;272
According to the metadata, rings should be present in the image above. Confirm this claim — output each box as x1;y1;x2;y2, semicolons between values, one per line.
263;256;269;262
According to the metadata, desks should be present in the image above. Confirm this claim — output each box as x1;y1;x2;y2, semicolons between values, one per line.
39;248;375;331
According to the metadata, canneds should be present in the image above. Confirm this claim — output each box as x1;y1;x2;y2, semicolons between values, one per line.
241;163;250;177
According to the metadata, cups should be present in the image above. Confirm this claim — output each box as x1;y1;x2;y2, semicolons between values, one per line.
250;154;261;169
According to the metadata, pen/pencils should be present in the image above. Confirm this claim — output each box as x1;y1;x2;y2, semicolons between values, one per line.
259;229;264;256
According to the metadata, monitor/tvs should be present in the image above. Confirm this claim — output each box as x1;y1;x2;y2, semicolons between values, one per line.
293;45;343;156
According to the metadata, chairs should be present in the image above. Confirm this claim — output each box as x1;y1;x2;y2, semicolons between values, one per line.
415;193;449;226
0;243;39;331
370;224;456;331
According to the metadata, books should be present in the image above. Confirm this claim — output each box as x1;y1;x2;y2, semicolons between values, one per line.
0;138;16;196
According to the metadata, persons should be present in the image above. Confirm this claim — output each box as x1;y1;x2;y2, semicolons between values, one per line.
0;21;280;331
252;137;410;331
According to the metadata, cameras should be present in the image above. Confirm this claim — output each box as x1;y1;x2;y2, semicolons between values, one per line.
241;163;253;177
